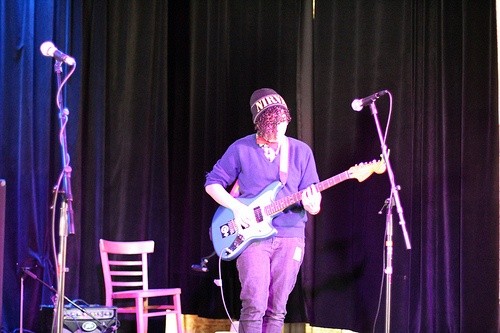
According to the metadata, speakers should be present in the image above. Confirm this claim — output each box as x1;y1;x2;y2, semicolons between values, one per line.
39;304;120;333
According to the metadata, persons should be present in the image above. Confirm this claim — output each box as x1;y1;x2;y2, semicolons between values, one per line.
204;88;322;333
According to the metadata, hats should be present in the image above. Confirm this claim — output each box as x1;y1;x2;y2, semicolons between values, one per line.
250;88;289;124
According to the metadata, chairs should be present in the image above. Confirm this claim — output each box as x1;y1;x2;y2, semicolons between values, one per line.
99;237;183;333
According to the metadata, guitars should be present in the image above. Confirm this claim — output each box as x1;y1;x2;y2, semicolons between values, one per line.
212;159;387;261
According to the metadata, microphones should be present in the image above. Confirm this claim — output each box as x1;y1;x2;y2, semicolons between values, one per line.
351;90;387;111
40;41;75;66
